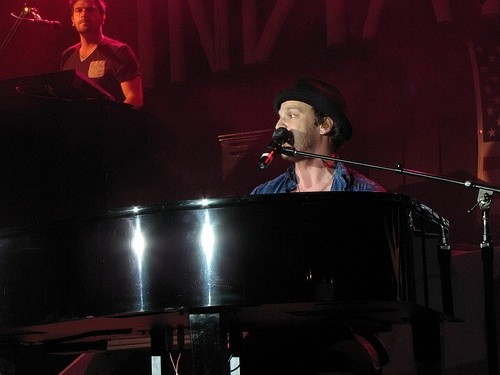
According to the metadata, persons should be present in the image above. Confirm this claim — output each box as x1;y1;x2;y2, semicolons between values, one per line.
61;0;143;110
227;85;393;375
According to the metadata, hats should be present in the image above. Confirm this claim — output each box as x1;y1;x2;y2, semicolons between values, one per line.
273;76;353;140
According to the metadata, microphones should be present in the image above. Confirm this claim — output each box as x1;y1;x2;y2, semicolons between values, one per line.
34;19;60;29
258;127;289;170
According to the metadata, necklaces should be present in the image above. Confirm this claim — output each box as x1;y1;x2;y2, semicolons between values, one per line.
79;34;103;60
295;168;336;192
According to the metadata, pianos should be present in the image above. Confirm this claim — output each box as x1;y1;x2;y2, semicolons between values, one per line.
0;191;466;375
